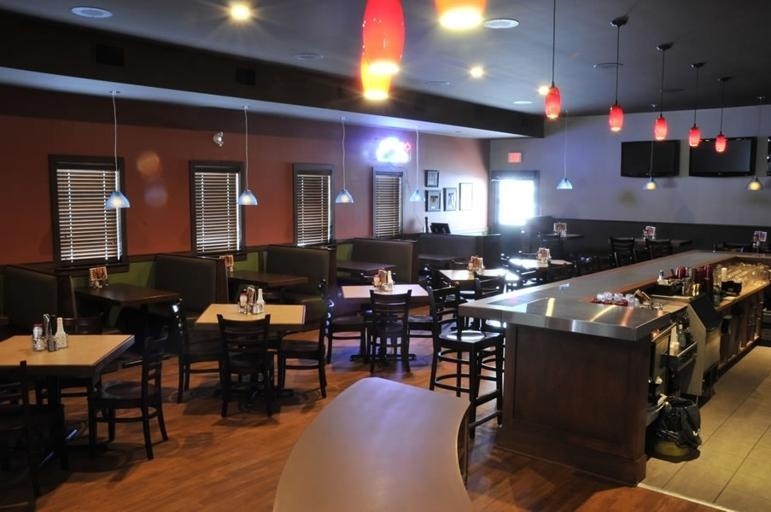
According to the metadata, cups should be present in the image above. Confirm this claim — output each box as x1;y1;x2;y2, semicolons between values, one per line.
713;261;771;286
592;291;639;308
677;263;713;282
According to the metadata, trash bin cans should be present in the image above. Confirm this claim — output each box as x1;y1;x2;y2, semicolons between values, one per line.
654;397;700;456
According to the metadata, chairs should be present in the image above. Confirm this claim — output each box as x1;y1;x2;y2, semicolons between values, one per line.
92;325;169;459
2;359;74;498
168;299;226;402
366;289;413;376
276;296;338;401
214;311;282;420
4;220;771;371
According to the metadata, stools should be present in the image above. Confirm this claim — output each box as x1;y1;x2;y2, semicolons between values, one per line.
426;286;506;439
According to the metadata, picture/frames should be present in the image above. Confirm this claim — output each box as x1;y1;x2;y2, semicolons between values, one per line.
424;189;442;213
442;186;458;212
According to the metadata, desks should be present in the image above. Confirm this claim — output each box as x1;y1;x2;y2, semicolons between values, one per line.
2;334;136;454
195;300;308;398
271;376;473;512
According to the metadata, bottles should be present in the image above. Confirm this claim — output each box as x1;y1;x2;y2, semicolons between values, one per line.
33;323;45;352
469;256;484;273
239;289;248;313
754;232;758;243
90;279;109;288
678;324;688;349
537;247;551;261
373;270;394;290
229;264;234;273
54;316;68;349
257;288;265;313
714;285;722;307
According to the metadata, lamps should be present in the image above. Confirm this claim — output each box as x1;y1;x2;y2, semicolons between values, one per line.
406;125;427;204
359;0;405;105
607;18;626;135
432;1;490;42
557;107;572;193
746;96;760;194
235;103;260;208
652;42;674;140
687;59;703;150
643;102;658;193
545;0;563;123
714;75;728;155
103;89;133;213
336;113;354;204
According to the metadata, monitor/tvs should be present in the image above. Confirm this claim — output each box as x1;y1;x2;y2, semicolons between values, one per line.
621;140;680;176
689;136;757;177
